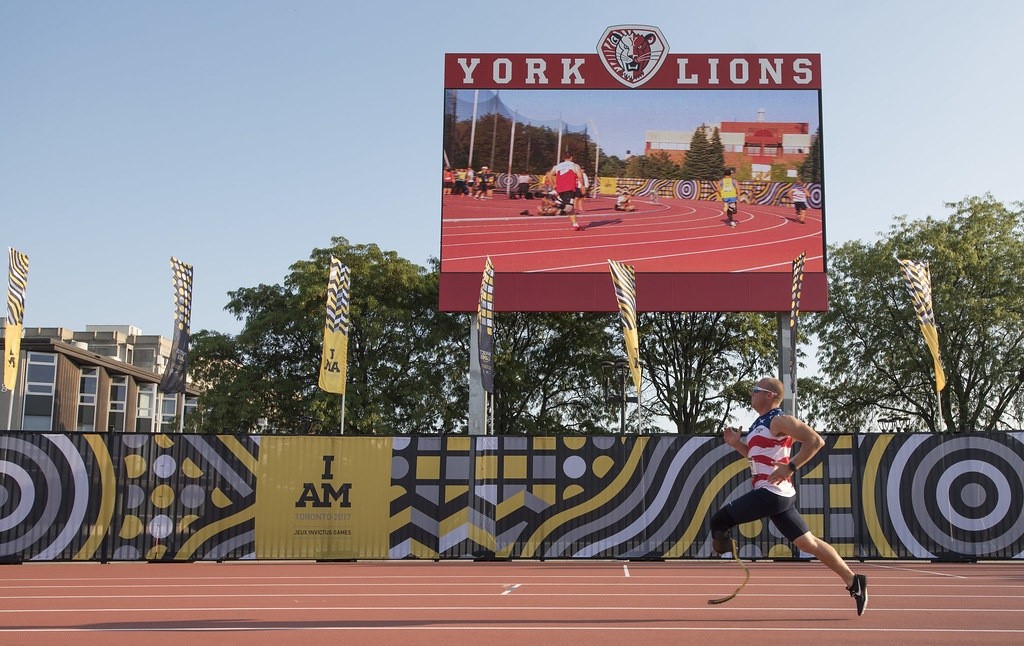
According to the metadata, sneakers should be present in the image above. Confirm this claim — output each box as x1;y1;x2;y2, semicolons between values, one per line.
844;574;868;617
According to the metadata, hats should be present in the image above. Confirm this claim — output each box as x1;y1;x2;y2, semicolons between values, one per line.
723;170;731;175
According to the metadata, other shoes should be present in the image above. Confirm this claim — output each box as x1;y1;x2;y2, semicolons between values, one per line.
573;225;582;231
732;224;736;228
724;202;728;212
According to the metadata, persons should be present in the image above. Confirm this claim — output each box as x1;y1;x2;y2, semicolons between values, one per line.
543;151;589;231
443;166;499;200
513;170;533;199
614;187;636;212
717;168;741;227
707;378;869;617
790;178;812;225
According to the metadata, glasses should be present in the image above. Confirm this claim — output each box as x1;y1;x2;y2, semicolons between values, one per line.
753;385;777;396
